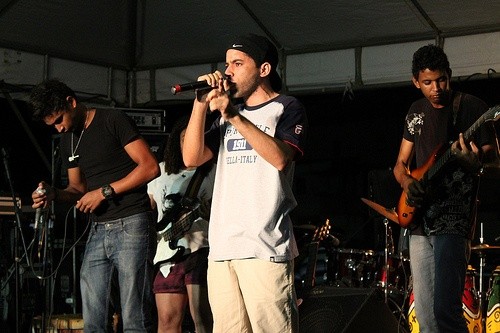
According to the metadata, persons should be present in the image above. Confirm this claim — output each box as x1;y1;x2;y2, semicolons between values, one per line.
182;32;306;333
28;80;161;333
393;45;500;333
147;116;217;333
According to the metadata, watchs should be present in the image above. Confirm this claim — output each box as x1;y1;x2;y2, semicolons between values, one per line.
101;184;114;201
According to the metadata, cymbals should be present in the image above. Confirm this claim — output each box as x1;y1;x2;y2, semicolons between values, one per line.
471;243;500;250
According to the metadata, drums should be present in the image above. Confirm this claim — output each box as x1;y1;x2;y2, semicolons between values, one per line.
330;242;500;333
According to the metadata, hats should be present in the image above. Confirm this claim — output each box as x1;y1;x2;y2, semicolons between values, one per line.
225;33;283;93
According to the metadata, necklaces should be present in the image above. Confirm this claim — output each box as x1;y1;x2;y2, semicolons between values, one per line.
68;107;89;162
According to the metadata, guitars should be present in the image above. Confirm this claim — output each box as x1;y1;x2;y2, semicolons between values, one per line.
361;198;400;225
152;199;213;265
396;103;500;231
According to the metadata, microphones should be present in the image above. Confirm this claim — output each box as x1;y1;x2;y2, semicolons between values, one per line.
33;185;48;238
328;235;340;246
170;76;228;96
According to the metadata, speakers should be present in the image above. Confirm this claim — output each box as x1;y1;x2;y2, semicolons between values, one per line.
299;288;403;333
